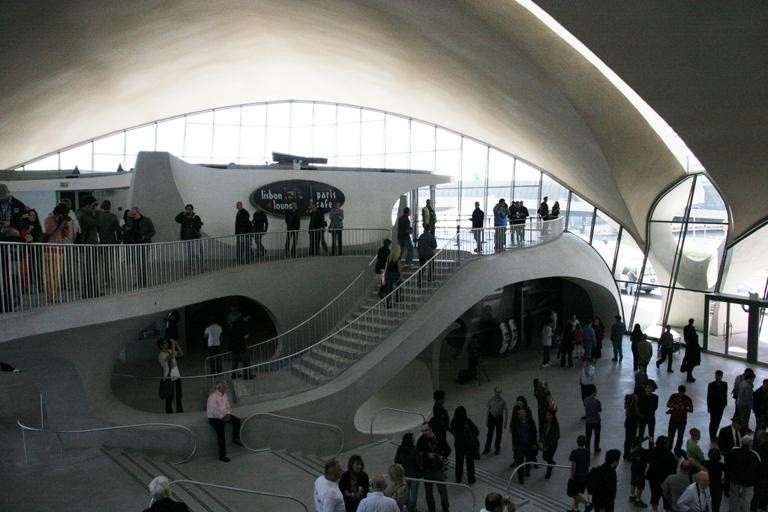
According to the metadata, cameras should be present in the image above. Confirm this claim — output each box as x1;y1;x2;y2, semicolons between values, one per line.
504;203;507;208
666;410;673;414
61;213;71;222
642;436;649;441
186;212;190;217
95;203;105;208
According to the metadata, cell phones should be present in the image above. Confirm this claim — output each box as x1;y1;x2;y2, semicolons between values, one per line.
169;338;175;343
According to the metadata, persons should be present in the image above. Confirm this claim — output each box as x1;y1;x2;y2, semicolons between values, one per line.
234;198;343;267
394;390;480;510
157;309;184;413
466;306;766;510
374;200;436;309
1;184;156;313
145;476;189;510
206;381;243;463
469;196;560;253
2;363;24;373
204;303;256;380
313;455;409;510
175;204;202;276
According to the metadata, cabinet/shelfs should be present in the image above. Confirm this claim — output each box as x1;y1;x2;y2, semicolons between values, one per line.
515;286;562;341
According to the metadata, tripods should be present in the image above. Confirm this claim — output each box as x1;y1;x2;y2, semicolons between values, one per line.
471;358;490;386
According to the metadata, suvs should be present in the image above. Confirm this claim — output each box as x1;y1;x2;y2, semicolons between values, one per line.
618;262;659;293
